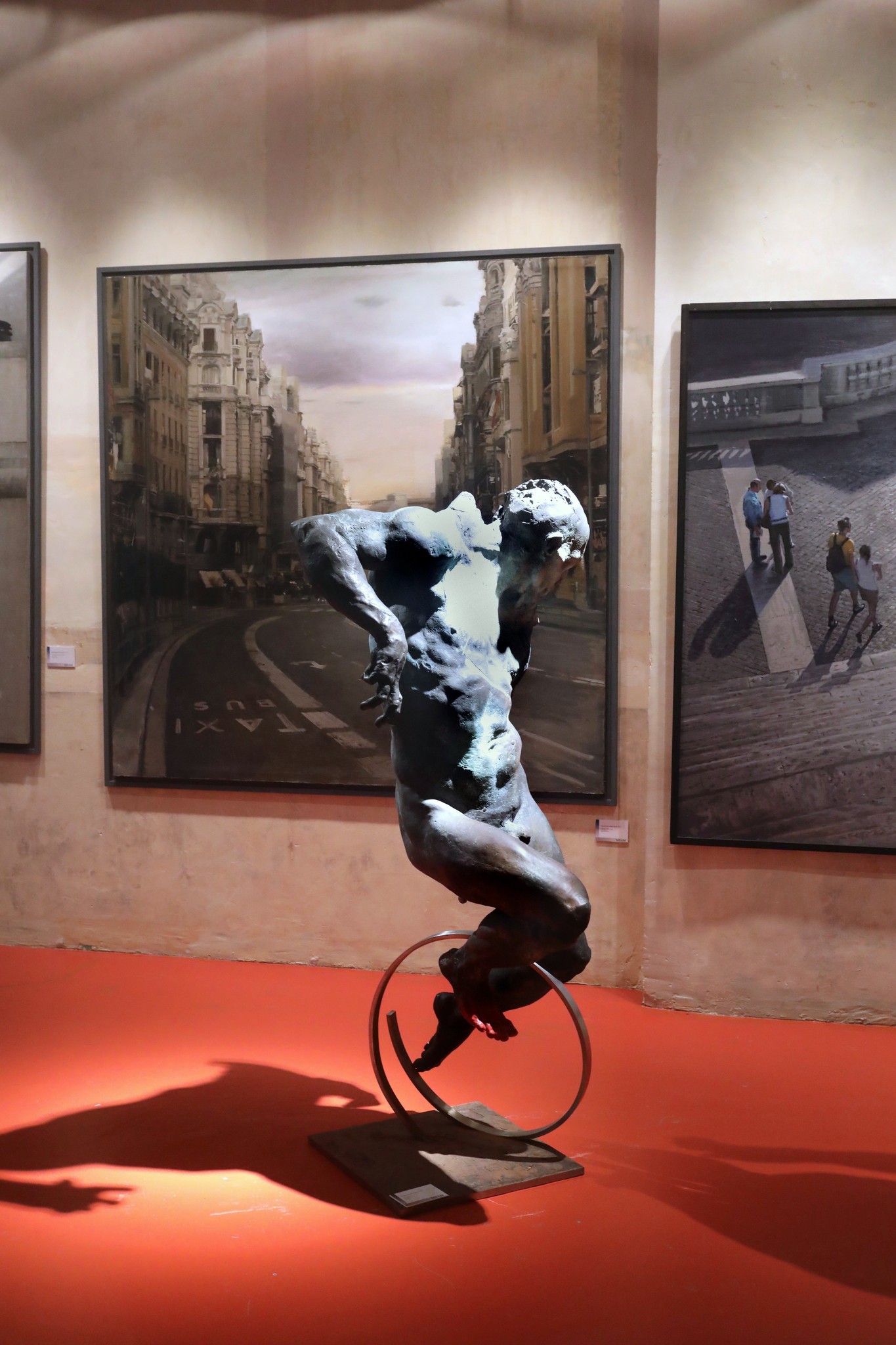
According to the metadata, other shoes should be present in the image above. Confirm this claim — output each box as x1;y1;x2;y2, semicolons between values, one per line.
828;619;838;627
853;603;865;614
872;622;883;633
753;555;767;561
755;561;768;567
792;543;794;547
856;632;862;644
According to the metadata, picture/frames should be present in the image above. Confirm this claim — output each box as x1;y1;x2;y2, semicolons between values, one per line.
0;240;50;758
95;238;623;808
666;295;896;860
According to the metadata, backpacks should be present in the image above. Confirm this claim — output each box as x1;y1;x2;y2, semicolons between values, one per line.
826;531;850;574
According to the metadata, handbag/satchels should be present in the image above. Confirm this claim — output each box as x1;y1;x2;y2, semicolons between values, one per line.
758;513;771;529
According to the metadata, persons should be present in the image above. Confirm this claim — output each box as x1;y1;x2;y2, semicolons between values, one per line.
764;479;795;549
827;517;866;627
761;483;794;574
286;474;594;1071
856;544;882;644
743;479;769;569
